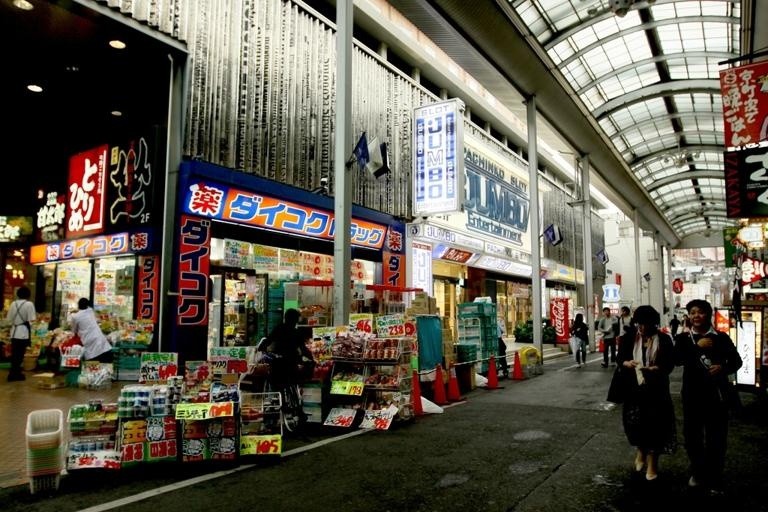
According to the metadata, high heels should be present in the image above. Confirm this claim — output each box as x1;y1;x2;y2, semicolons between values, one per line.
634;456;657;481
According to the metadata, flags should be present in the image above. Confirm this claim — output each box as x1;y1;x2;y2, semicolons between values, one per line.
643;273;650;281
353;132;391;179
597;250;609;265
543;224;563;246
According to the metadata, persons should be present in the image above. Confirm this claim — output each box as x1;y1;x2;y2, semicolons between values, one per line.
669;315;680;339
607;306;675;481
8;286;36;381
271;307;317;389
71;298;112;362
674;300;742;496
680;314;692;334
598;306;634;367
571;313;589;368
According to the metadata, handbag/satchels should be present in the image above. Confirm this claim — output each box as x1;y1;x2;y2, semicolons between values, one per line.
606;367;628;405
623;326;631;332
715;382;746;422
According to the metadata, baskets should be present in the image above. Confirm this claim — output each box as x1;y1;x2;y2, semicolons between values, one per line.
25;409;65;494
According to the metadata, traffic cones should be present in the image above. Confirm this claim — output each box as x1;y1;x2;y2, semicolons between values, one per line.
433;360;461;405
599;339;603;353
487;355;498;390
412;370;422;417
512;351;523;380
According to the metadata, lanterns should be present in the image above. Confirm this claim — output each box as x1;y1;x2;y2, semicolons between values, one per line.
672;279;683;294
